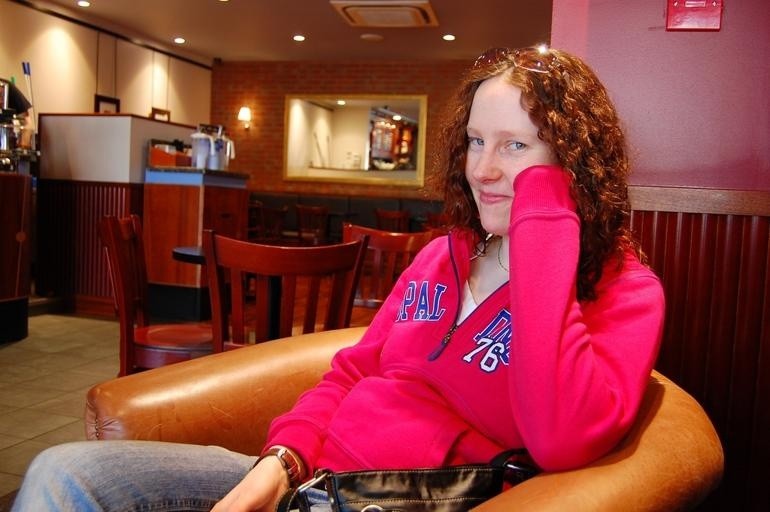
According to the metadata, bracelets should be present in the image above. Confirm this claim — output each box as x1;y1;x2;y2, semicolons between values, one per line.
249;447;303;491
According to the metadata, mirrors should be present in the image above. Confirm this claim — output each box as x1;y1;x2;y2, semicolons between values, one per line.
281;95;428;188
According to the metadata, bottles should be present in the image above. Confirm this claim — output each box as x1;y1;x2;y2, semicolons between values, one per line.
18;155;31;174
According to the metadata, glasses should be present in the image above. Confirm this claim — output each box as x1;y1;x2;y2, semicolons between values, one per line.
471;46;569;82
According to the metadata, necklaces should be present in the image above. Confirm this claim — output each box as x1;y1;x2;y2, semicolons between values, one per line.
497;241;509;273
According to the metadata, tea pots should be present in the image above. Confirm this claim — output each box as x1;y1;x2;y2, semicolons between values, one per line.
190;130;236;171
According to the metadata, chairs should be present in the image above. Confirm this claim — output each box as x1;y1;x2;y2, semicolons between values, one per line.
83;325;725;512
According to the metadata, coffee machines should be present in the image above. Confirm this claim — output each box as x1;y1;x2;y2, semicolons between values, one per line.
1;78;32;171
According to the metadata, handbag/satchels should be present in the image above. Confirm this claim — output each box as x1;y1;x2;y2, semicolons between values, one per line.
315;464;506;512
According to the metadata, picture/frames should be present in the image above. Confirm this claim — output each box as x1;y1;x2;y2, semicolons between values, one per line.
93;94;120;114
151;107;170;122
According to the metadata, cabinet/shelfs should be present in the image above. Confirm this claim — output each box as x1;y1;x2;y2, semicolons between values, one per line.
142;166;250;326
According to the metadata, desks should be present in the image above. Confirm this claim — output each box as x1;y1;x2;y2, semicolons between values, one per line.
0;172;33;347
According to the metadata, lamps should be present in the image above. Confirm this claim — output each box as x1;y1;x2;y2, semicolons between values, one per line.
236;107;251;129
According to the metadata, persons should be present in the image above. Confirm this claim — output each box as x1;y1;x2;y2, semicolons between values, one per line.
9;45;666;512
